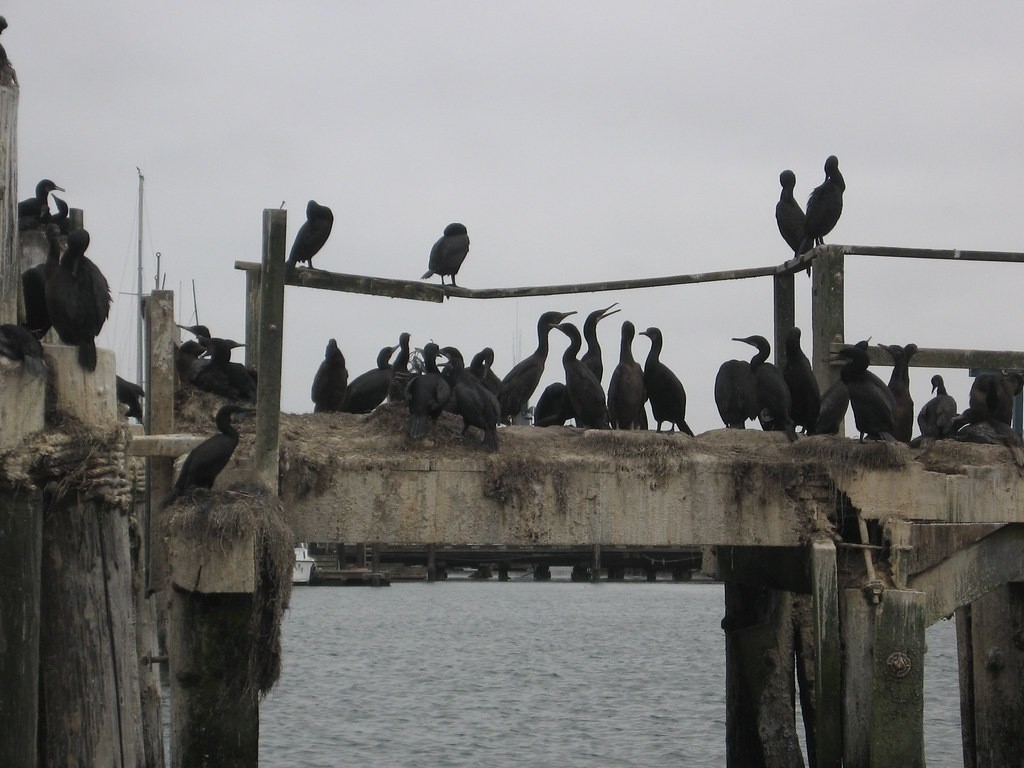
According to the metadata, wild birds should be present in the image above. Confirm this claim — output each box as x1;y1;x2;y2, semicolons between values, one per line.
776;156;845;273
0;178;254;510
537;304;872;441
288;200;576;446
823;339;1022;454
